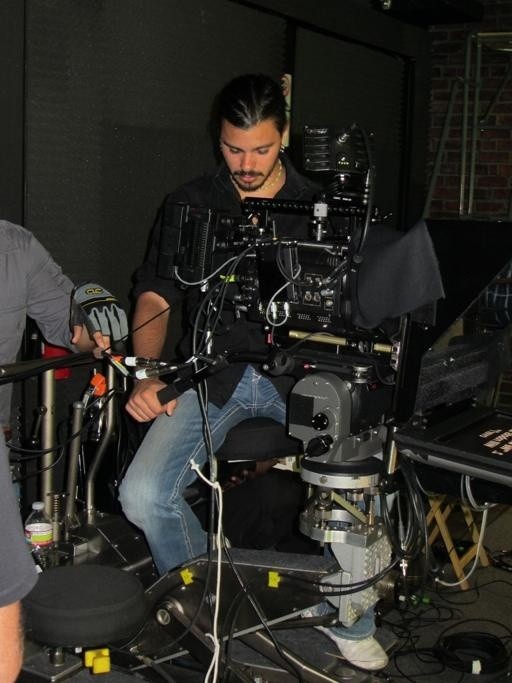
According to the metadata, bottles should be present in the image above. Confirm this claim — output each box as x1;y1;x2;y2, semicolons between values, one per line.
24;502;56;571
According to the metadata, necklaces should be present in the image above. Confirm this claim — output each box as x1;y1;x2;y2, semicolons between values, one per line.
256;158;283;192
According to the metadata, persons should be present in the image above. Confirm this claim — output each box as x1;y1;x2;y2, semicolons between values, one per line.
0;217;130;683
118;73;390;672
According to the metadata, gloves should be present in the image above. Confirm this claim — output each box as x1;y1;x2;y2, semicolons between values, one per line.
71;279;129;342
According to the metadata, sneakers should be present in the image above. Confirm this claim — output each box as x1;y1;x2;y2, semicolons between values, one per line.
301;607;389;671
